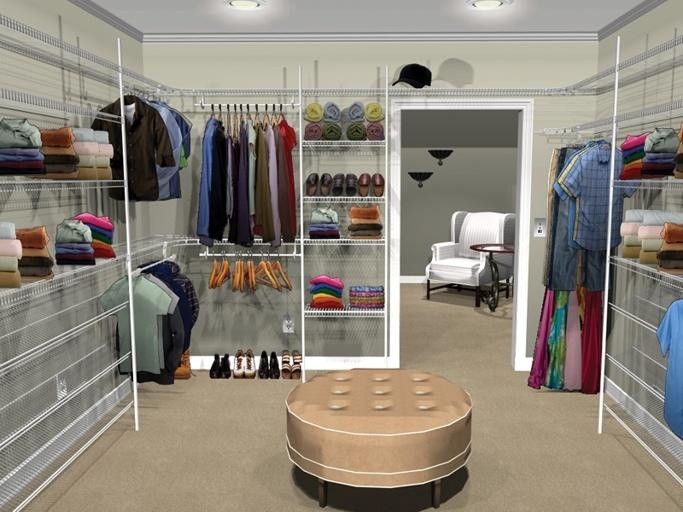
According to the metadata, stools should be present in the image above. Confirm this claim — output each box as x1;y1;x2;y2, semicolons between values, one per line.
286;368;474;507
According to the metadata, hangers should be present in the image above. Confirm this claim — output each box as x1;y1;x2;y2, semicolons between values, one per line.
205;102;289;140
209;251;293;294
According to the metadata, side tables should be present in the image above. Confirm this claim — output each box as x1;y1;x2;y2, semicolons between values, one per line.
470;242;516;311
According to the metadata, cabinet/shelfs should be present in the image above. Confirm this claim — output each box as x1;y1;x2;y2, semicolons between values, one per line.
598;33;683;504
2;36;143;512
300;86;393;367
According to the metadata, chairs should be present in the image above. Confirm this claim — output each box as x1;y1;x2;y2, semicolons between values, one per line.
426;210;517;307
